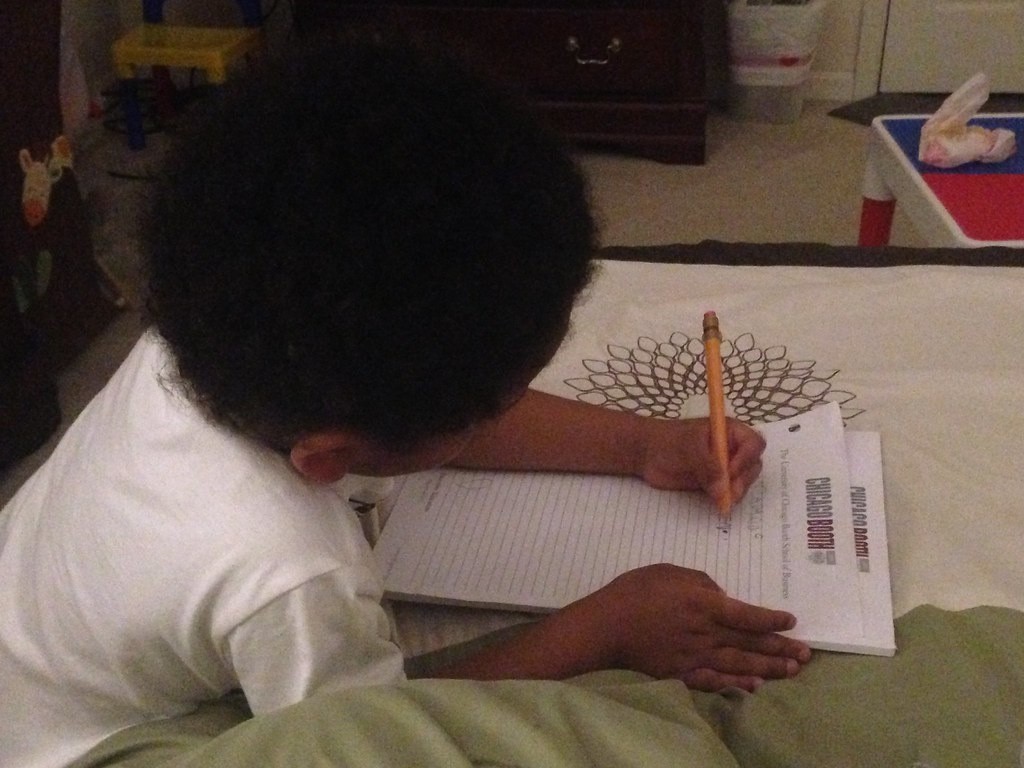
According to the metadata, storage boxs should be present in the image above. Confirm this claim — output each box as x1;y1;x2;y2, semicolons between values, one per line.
721;1;822;124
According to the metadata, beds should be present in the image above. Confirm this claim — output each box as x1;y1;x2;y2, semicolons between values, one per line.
50;233;1021;767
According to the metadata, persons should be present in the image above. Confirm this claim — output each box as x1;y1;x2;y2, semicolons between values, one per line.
1;20;817;768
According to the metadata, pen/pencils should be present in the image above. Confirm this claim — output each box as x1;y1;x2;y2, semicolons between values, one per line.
702;311;733;522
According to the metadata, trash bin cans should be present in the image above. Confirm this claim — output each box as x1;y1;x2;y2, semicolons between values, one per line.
729;0;822;123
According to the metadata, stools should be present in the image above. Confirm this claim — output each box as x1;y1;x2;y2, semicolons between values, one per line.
852;110;1024;243
113;24;264;156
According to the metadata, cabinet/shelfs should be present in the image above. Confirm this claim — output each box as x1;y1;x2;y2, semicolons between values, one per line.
510;0;733;166
